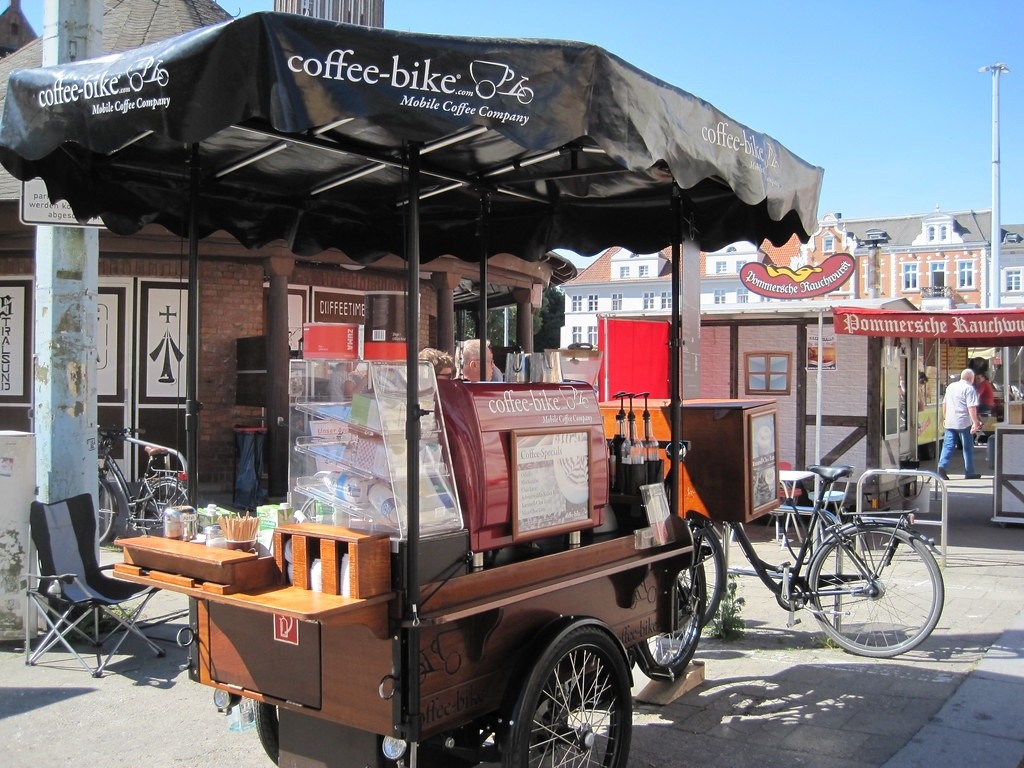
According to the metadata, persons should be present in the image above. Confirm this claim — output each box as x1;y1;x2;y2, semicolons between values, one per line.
937;368;981;481
975;374;994;426
418;339;504;384
329;355;369;403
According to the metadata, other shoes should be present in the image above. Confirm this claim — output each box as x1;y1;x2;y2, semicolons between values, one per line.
937;467;949;480
965;473;981;479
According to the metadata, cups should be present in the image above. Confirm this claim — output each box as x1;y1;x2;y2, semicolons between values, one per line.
226;539;257;554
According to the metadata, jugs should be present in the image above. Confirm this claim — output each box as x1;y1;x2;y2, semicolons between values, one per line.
505;352;531;382
529;352;563;384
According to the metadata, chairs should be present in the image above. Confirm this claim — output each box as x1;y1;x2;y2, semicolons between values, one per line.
764;460;855;538
24;493;166;678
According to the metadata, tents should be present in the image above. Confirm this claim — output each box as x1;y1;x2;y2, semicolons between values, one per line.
0;12;827;269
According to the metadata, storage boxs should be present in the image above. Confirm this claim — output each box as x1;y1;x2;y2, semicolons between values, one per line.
1009;401;1024;424
303;322;364;360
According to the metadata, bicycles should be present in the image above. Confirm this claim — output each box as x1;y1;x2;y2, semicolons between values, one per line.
94;423;190;540
682;463;945;658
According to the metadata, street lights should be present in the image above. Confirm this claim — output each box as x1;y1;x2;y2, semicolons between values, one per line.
979;60;1012;306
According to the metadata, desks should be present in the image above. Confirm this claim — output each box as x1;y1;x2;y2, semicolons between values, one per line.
729;470;814;550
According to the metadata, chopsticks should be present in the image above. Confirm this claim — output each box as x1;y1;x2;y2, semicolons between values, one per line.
218;511;260;538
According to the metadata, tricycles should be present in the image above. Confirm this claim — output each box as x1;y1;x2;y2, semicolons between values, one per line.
1;10;825;763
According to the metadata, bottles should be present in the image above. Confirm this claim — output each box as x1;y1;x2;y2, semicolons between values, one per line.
642;417;659;462
323;471;367;502
605;415;628;496
622;421;643;465
370;484;406;525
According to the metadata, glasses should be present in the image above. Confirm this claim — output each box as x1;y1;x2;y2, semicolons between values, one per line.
437;372;453;378
